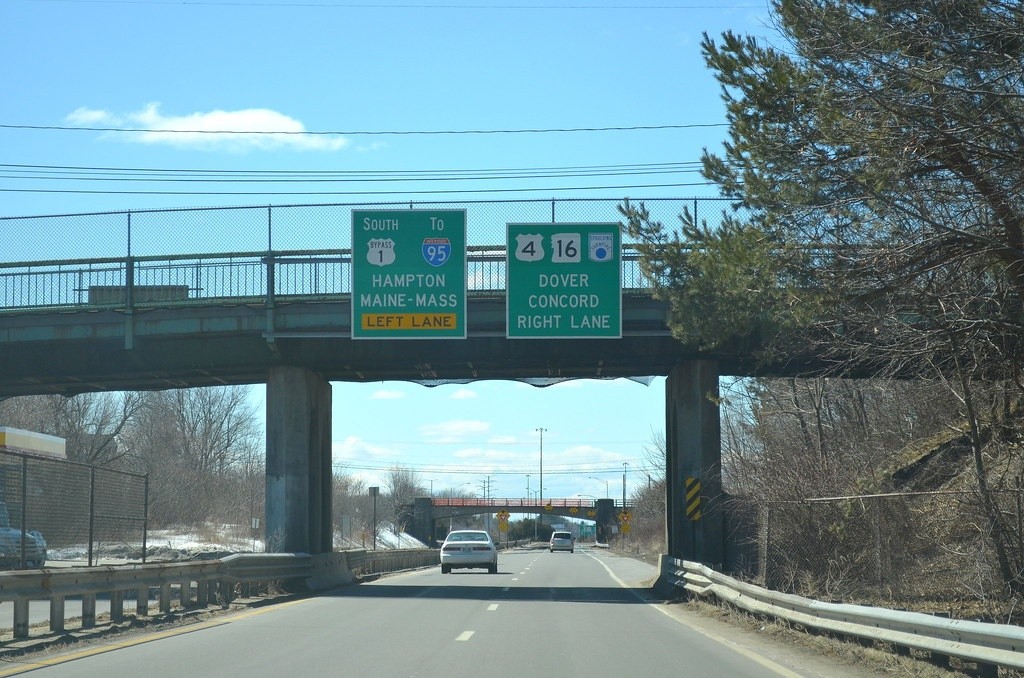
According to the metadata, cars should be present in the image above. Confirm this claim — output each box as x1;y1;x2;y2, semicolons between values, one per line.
440;529;499;575
549;531;576;554
0;503;47;570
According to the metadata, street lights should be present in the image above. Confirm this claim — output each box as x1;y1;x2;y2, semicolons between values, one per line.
588;476;609;497
525;487;548;506
535;427;548;524
623;462;629;474
451;482;470;497
